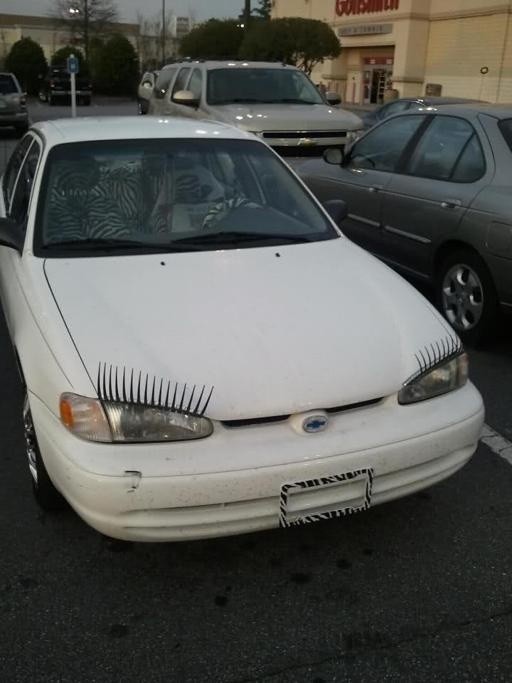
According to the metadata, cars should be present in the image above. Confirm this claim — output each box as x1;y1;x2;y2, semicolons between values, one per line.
136;68;161;115
0;112;488;551
360;94;492;130
285;99;511;339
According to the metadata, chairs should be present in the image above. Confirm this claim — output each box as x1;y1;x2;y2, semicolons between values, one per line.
147;152;249;238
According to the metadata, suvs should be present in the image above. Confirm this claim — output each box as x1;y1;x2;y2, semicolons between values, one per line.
145;53;366;159
0;70;32;136
37;63;94;106
